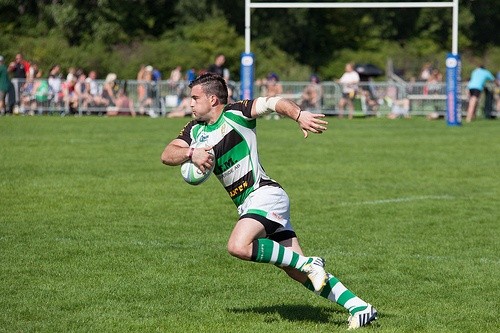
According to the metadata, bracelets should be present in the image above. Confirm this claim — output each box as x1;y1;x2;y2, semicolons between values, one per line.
296;109;303;123
189;148;194;159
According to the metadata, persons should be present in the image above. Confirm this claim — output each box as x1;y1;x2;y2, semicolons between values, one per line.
161;72;378;330
0;52;500;120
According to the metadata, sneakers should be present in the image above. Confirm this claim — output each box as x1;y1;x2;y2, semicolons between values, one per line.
300;257;328;292
346;302;378;331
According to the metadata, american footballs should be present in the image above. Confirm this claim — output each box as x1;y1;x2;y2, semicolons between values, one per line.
181;142;217;185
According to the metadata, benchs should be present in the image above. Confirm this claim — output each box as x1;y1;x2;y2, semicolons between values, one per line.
407;95;470;112
281;94;367;111
